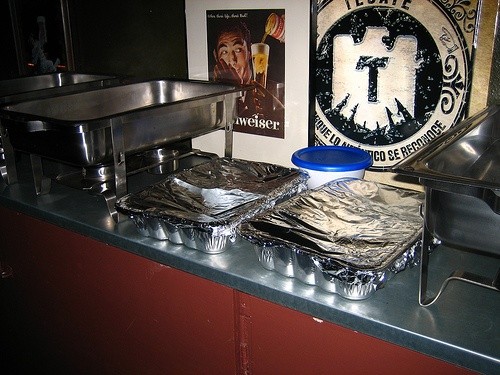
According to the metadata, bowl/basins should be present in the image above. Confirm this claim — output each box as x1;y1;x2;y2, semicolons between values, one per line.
293;147;372;190
145;149;180;174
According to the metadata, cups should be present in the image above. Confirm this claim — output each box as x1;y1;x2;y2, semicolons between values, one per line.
265;14;284;43
251;43;270;119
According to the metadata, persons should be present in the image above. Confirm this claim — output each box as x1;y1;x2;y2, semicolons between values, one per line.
209;17;284;139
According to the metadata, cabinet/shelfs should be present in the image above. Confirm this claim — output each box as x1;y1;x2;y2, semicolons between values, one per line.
0;198;499;374
